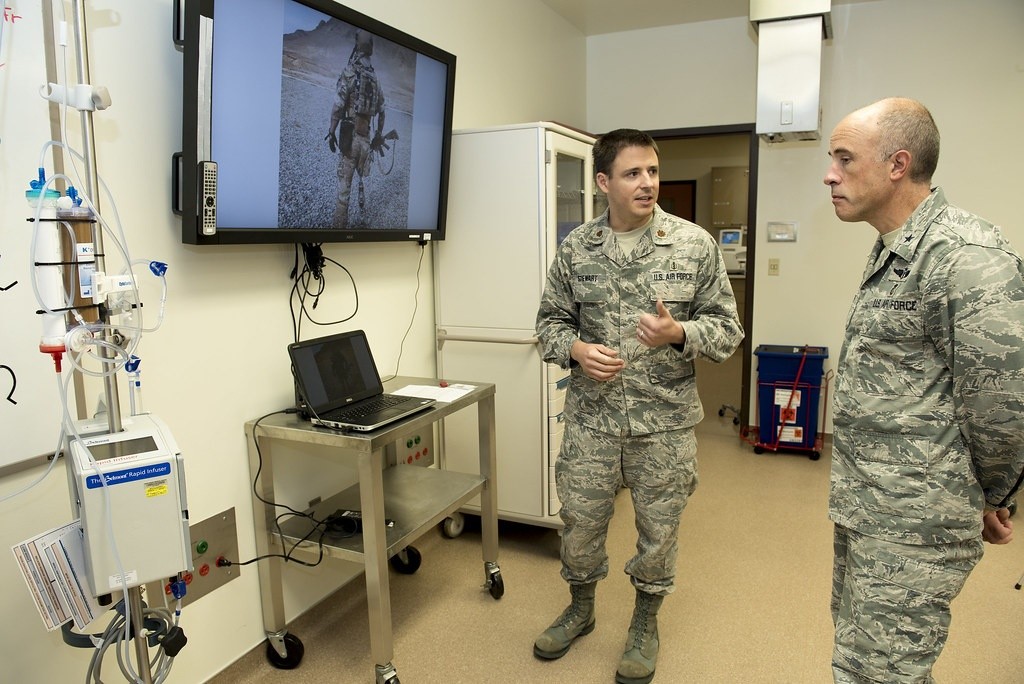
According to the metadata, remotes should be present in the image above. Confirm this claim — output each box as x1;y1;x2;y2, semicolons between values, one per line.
198;160;218;236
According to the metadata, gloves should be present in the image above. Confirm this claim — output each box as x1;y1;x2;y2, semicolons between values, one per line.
324;128;338;152
370;132;382;149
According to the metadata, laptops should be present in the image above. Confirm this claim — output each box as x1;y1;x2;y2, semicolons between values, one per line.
287;329;437;431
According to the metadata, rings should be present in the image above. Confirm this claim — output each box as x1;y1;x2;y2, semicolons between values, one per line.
640;331;645;338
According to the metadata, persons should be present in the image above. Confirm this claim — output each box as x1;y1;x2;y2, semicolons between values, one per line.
532;129;744;684
329;31;386;229
824;97;1024;684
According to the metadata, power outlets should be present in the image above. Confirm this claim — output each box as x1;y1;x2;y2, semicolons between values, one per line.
147;509;241;621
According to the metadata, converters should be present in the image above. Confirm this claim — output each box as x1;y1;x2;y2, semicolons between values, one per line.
330;508;363;531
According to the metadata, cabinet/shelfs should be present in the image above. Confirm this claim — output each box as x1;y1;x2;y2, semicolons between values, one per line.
244;376;504;684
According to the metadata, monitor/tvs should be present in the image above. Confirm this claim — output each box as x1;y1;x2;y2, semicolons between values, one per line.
173;0;457;245
719;230;742;251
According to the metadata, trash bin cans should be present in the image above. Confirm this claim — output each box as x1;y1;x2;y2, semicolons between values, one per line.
755;344;829;449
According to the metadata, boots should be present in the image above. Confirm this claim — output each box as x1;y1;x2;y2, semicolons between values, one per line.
615;589;664;684
534;581;597;659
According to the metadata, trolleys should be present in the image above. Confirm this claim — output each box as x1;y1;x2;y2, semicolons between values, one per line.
245;374;506;684
740;368;835;461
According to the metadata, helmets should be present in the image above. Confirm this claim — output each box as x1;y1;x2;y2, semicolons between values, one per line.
355;30;373;55
